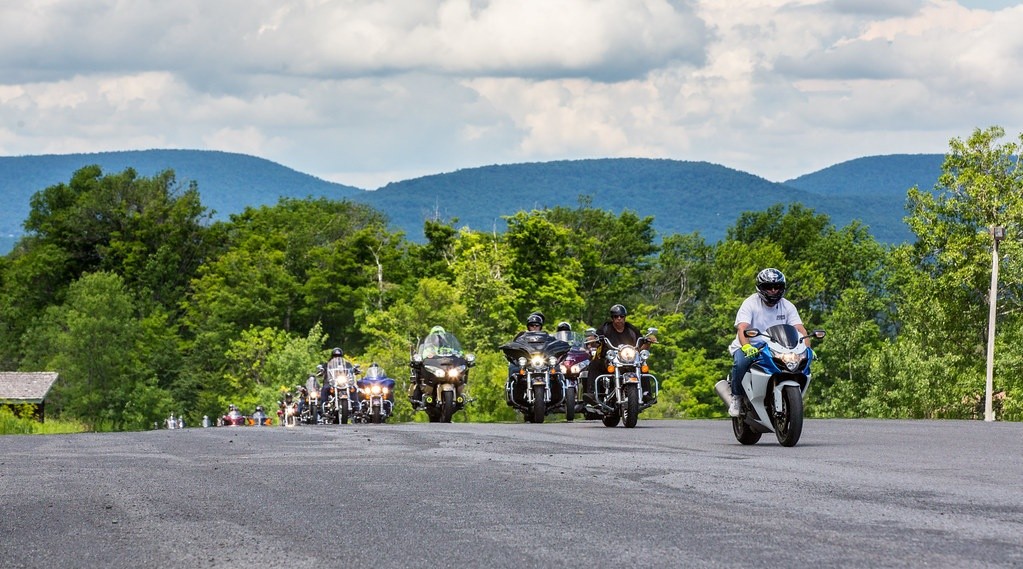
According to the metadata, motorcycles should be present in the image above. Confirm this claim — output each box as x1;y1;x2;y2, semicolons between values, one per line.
357;367;395;423
166;417;212;430
409;330;473;422
317;357;362;425
224;410;244;426
251;411;265;426
281;394;316;426
580;327;660;428
548;331;590;423
713;326;835;446
499;332;572;423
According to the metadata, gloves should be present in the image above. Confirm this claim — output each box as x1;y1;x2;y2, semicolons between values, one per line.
810;348;817;360
741;344;760;358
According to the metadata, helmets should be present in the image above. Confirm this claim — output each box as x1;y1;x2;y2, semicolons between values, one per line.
332;348;343;355
526;312;545;326
229;404;234;407
430;325;446;334
370;363;378;367
286;393;292;397
610;304;627;315
256;407;261;410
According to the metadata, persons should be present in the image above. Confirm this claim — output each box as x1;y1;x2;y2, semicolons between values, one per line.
729;268;817;417
164;347;396;428
413;325;465;409
505;304;657;409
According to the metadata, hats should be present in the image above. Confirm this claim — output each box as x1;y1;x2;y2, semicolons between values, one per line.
558;322;570;331
755;268;786;303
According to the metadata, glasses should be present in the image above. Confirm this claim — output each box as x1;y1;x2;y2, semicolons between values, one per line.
764;285;780;291
528;324;540;327
612;315;626;319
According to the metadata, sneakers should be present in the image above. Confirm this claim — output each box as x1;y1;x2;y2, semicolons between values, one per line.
728;395;742;417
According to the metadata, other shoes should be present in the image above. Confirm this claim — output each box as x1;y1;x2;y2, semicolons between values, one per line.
317;409;327;414
582;393;596;406
643;393;657;404
352;407;359;414
